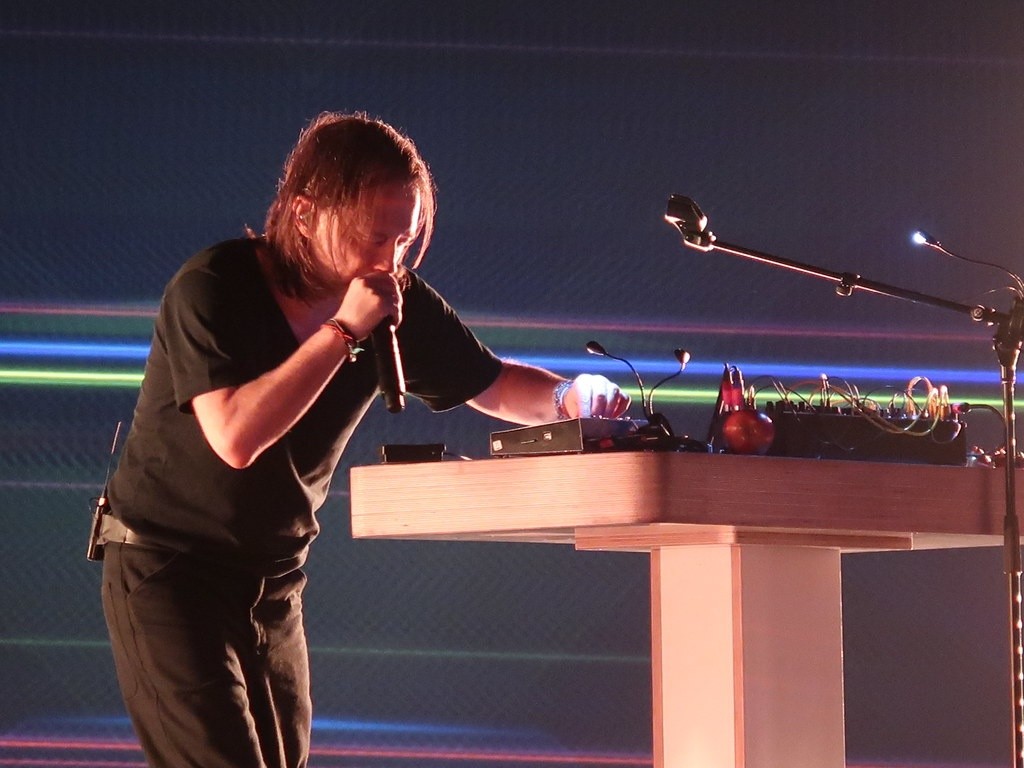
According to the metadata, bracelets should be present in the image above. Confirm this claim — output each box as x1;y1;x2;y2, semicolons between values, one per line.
320;318;360;363
553;379;574;420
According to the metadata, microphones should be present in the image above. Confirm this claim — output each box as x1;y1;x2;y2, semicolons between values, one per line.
371;313;405;414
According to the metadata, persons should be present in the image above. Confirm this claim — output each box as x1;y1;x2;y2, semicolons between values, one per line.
100;110;631;768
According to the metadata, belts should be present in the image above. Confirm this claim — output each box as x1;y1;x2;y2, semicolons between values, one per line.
100;512;172;548
